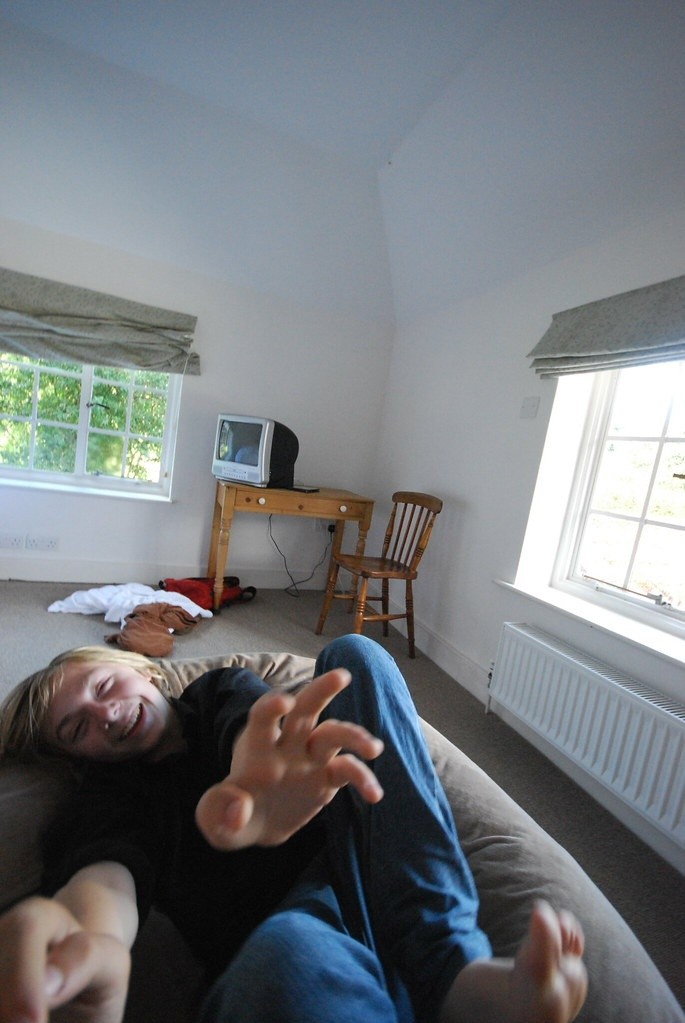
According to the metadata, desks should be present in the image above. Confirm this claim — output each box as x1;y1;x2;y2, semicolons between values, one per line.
208;478;375;612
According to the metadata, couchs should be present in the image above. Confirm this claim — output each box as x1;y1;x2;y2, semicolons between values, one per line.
1;654;682;1023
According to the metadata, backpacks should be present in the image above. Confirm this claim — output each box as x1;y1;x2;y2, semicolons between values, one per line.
159;575;257;610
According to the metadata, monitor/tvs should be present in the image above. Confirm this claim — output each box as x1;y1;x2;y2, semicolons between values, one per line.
210;414;299;489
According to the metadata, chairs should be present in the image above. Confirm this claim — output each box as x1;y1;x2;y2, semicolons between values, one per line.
316;491;443;659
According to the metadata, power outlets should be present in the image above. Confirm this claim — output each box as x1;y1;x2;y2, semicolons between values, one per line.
327;519;336;538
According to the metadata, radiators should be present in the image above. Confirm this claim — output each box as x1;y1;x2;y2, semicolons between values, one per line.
486;620;683;870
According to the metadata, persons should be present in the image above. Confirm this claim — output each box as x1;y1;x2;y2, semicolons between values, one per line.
1;634;587;1022
